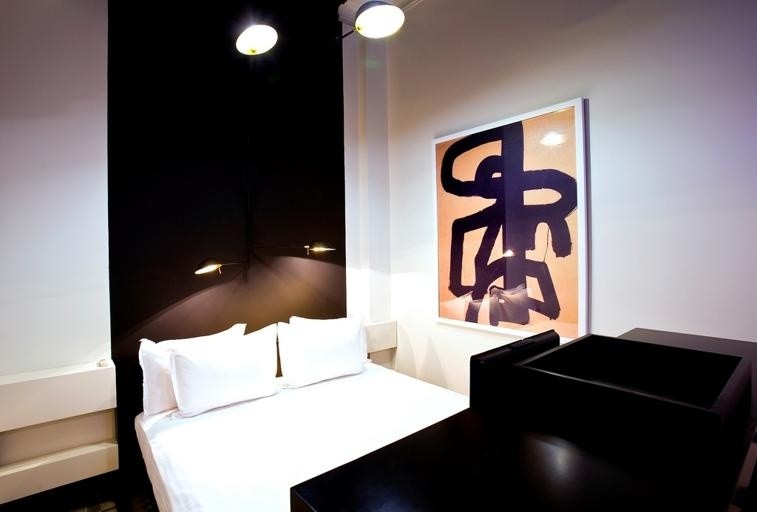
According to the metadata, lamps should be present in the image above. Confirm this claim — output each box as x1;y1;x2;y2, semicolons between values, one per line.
229;1;405;59
193;238;336;278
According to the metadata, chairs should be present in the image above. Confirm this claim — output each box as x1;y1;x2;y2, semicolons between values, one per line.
466;325;561;415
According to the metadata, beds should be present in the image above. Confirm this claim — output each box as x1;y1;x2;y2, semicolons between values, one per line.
131;359;468;512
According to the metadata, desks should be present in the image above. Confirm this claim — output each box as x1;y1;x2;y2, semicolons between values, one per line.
287;320;757;512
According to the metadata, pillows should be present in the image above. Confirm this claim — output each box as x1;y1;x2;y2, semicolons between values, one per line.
134;315;371;423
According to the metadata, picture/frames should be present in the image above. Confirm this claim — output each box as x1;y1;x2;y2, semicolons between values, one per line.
429;96;594;346
